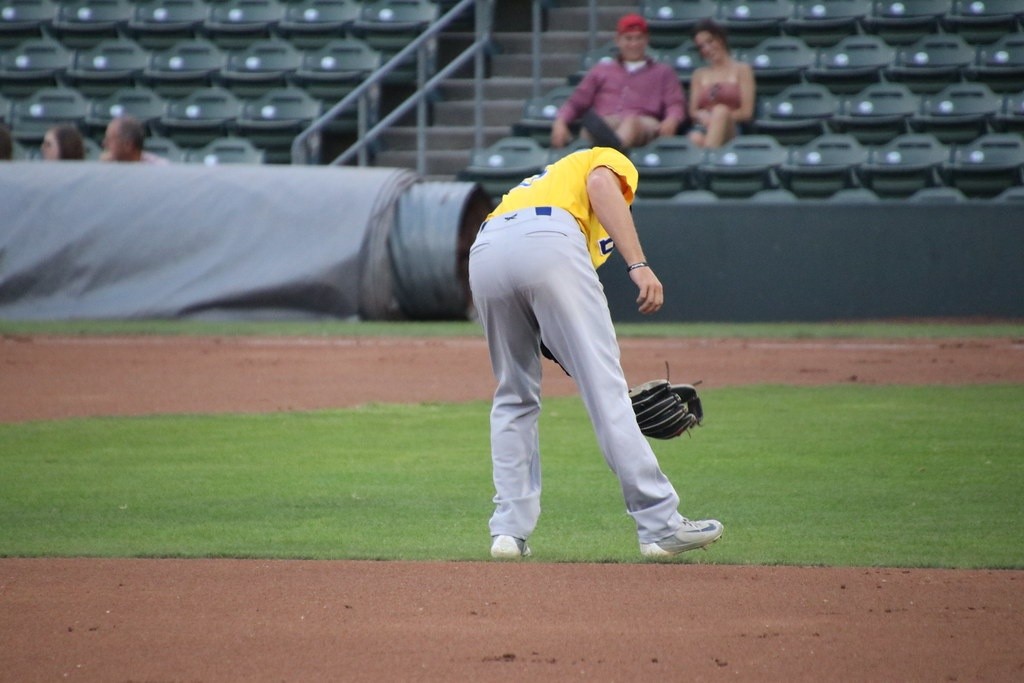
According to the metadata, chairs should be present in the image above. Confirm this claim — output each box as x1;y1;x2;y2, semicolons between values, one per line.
1;1;1024;183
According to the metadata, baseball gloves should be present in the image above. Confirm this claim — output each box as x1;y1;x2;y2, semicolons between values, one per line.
630;360;706;440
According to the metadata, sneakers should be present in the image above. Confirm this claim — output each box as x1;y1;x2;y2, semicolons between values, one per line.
640;515;725;559
491;534;530;560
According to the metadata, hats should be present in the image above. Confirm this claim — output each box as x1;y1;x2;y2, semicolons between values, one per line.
618;15;648;35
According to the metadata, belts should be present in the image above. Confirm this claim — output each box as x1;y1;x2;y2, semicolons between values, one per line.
480;205;552;231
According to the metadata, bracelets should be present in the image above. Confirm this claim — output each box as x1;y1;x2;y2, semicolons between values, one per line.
627;262;650;273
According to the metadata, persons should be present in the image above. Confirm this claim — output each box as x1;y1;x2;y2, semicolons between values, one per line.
687;18;755;146
552;13;685;150
468;145;723;560
98;115;171;165
40;126;86;161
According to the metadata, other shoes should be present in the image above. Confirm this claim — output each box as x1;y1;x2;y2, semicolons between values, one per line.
584;110;625;153
